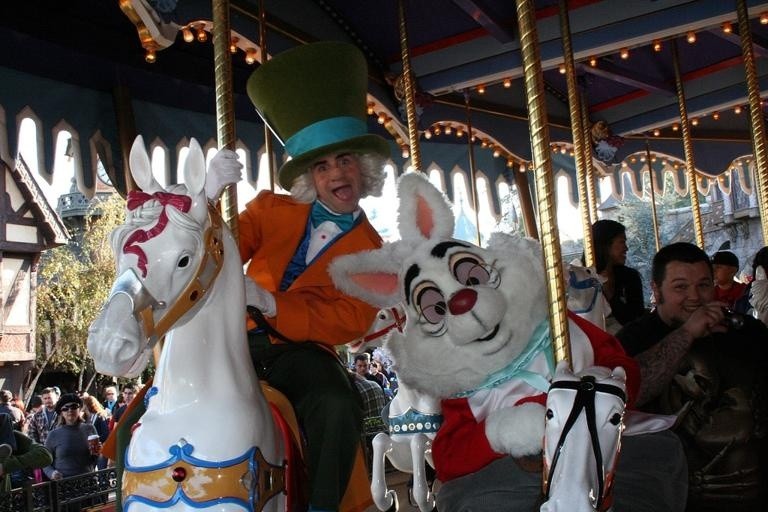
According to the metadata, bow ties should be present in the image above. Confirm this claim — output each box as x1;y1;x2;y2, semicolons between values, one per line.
311;200;354;233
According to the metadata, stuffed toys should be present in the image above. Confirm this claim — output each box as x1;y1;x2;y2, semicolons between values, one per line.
327;172;641;482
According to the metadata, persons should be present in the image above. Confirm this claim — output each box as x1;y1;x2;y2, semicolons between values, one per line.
742;246;768;315
709;251;747;310
0;383;139;512
580;219;644;325
224;144;383;512
352;351;398;396
616;241;767;413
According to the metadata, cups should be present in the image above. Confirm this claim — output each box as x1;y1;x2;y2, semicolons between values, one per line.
88;435;100;455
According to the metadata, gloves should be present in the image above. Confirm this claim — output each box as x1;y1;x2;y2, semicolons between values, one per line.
204;149;243;200
244;274;277;319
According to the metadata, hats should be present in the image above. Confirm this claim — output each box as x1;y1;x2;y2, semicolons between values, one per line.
246;40;392;192
710;251;739;272
54;393;83;415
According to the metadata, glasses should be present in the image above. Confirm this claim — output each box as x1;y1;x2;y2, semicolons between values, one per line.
60;405;80;412
